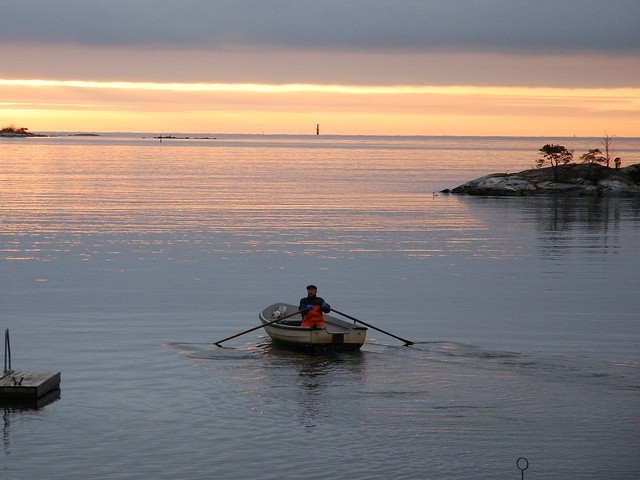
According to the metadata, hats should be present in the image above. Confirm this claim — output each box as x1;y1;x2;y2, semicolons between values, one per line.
306;285;317;290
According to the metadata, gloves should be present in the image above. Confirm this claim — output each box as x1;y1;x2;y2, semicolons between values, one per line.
322;303;329;308
307;305;313;310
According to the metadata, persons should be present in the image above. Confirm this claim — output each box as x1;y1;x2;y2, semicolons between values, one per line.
298;285;330;328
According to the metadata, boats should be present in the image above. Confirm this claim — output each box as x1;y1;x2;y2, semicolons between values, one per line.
215;303;415;351
